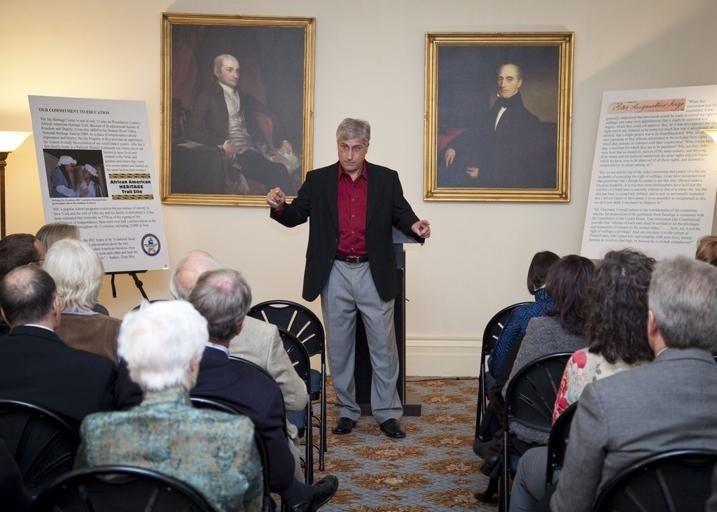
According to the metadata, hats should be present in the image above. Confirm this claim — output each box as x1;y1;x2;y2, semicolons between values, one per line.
58;156;77;166
83;164;98;177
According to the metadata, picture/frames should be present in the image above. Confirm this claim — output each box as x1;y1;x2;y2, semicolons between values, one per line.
420;28;576;211
155;9;318;212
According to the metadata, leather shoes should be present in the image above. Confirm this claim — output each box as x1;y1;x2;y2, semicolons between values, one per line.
380;418;406;437
281;476;338;512
333;417;357;434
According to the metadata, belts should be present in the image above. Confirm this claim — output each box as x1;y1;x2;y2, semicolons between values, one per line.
335;254;368;264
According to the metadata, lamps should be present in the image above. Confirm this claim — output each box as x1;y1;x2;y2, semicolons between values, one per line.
0;124;32;237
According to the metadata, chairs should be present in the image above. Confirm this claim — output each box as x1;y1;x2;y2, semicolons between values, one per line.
0;293;334;512
467;299;717;508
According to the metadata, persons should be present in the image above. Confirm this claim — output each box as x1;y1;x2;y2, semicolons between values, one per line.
76;163;99;198
77;248;339;511
0;223;144;510
472;235;717;512
438;61;548;188
265;117;430;439
51;155;89;197
186;53;291;196
